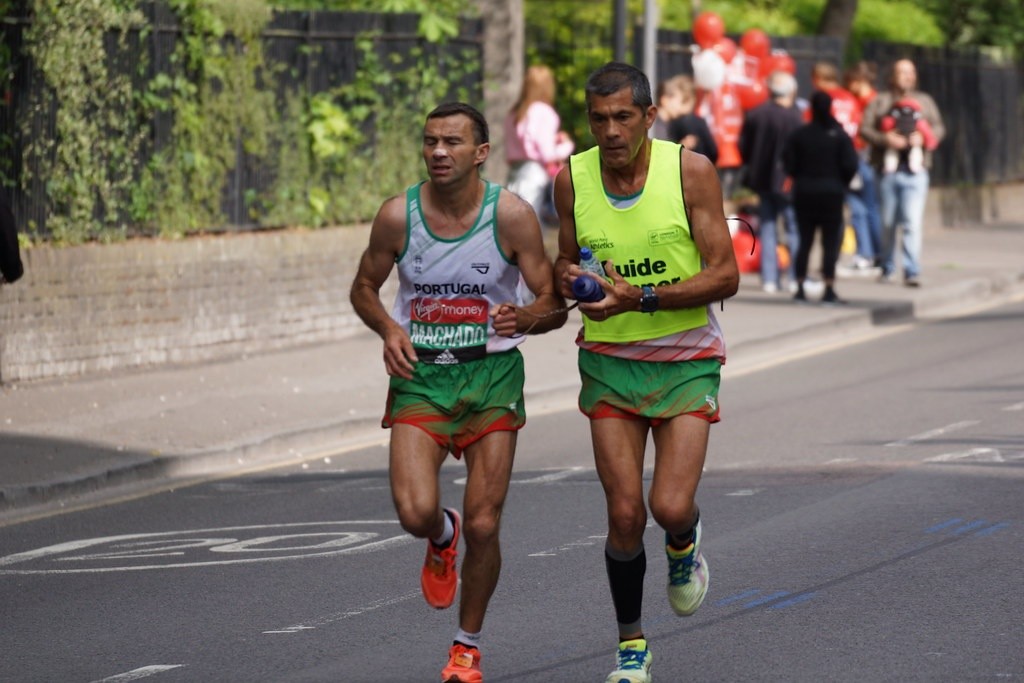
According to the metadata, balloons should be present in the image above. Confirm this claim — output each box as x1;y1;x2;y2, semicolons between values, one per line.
691;11;797;112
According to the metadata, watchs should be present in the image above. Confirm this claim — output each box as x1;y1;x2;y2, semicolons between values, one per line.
640;285;659;312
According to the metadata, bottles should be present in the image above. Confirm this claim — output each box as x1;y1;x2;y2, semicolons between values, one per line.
572;275;605;303
579;247;607;281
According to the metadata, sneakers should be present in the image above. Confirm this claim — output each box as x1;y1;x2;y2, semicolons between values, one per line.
420;508;461;610
442;640;483;682
665;518;709;617
606;639;652;683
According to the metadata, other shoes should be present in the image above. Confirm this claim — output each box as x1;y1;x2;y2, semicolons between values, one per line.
763;280;778;292
822;285;838;300
852;257;871;268
904;276;920;287
794;284;806;298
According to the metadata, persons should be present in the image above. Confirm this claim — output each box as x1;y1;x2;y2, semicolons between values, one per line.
349;102;568;683
504;59;946;308
553;61;740;683
